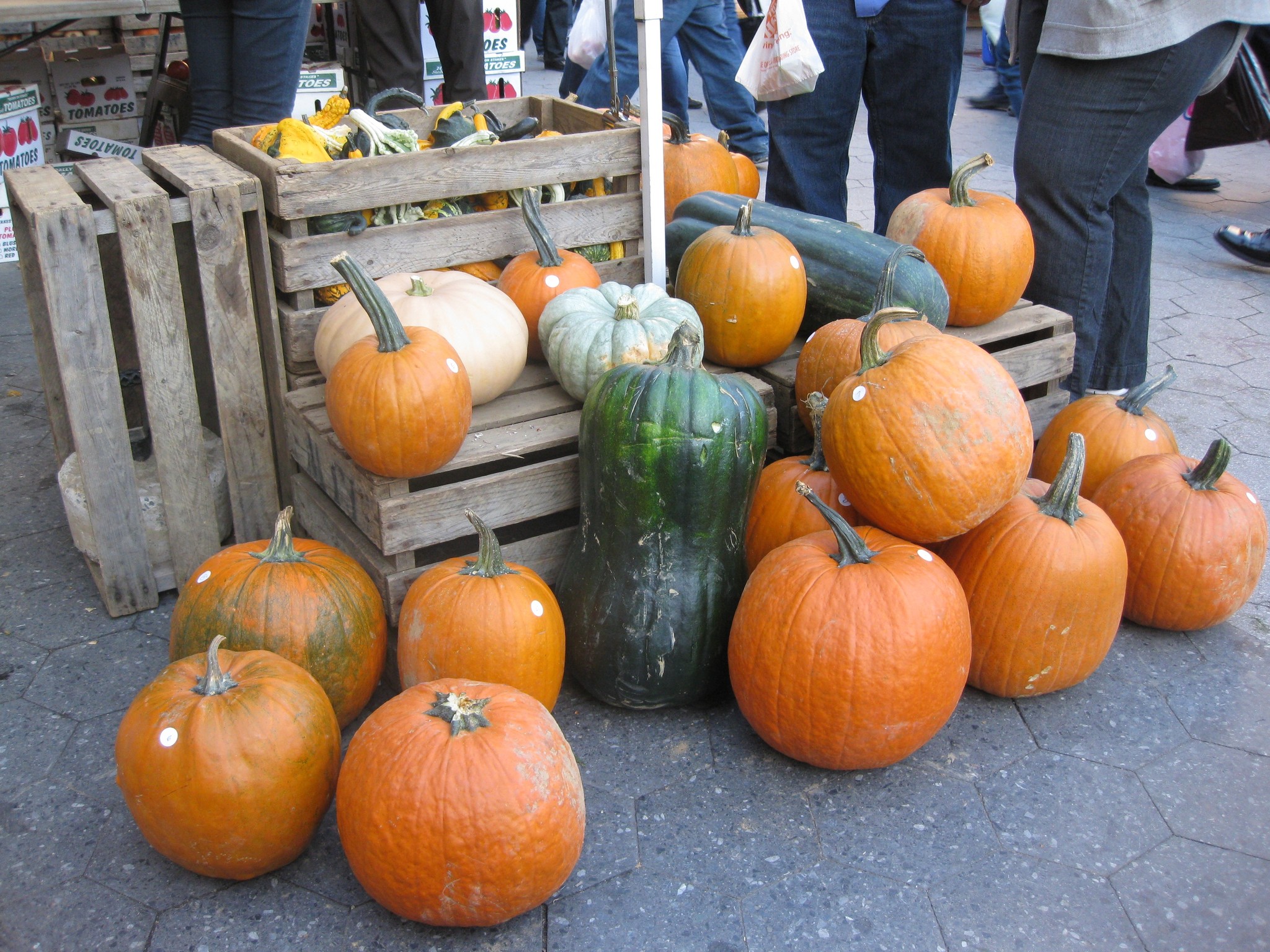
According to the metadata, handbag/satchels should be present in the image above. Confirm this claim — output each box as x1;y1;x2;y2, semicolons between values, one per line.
735;0;825;102
1148;98;1207;184
567;0;618;70
1185;38;1270;151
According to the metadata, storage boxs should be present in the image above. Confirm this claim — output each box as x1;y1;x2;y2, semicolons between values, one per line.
0;0;1076;634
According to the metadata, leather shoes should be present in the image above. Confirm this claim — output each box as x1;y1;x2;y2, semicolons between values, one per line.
1213;224;1270;267
1145;173;1221;191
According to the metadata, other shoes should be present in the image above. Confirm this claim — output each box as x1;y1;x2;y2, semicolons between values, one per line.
688;97;702;109
537;54;545;62
969;85;1011;108
544;59;566;72
752;156;769;164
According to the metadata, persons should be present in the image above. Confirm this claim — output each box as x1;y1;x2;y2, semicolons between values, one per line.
968;0;1270;122
1014;0;1270;401
179;0;313;148
355;0;488;112
519;0;992;237
1211;224;1269;271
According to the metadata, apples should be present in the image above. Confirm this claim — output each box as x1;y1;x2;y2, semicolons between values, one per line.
167;60;189;81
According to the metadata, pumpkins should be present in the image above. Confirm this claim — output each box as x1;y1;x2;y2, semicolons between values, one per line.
313;271;528;407
593;98;1034;369
495;186;602;365
322;250;473;478
729;246;1270;774
134;27;184;38
169;507;389;731
396;508;567;721
336;682;586;929
538;280;705;403
551;325;775;712
116;634;340;882
253;85;625;303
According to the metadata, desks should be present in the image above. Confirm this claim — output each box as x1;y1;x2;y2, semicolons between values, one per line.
0;0;373;147
